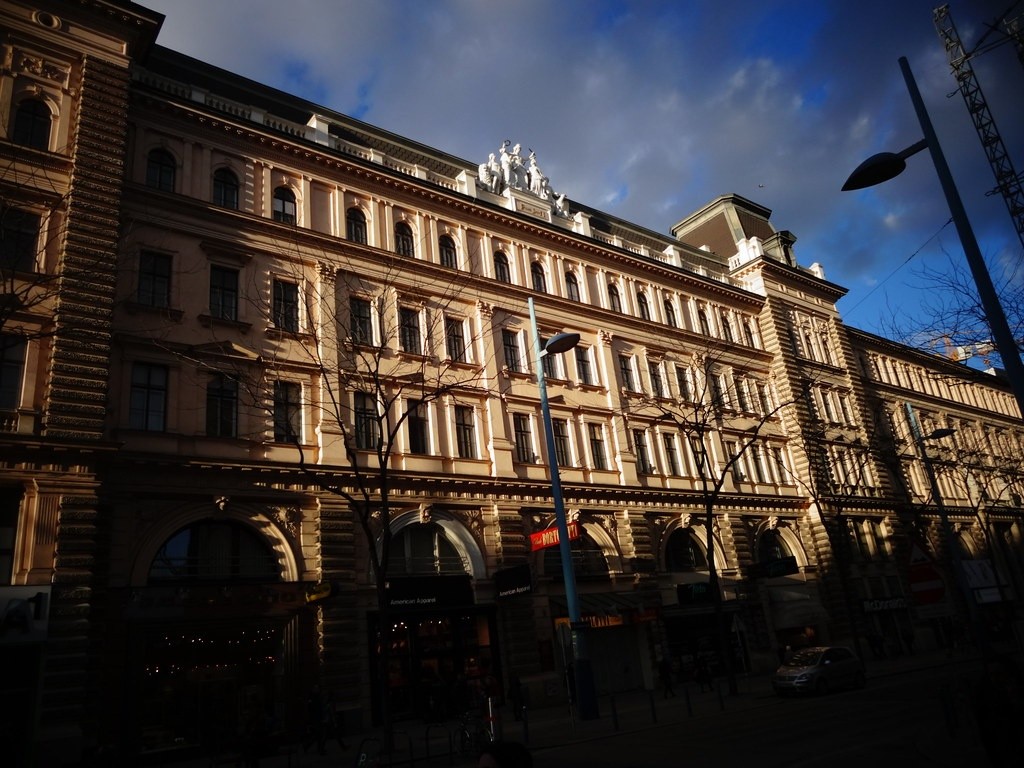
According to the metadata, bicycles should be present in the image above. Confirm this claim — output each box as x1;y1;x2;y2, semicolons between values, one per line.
452;711;496;755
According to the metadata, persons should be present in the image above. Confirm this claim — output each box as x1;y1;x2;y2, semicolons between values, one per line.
476;741;533;768
865;614;1024;662
784;645;793;661
657;654;715;701
487;143;552;201
507;670;525;721
303;685;351;756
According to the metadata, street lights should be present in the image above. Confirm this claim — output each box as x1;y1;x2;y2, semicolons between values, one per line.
915;426;978;641
525;294;599;722
839;55;1024;425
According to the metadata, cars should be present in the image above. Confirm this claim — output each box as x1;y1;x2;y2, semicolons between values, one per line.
773;645;866;697
